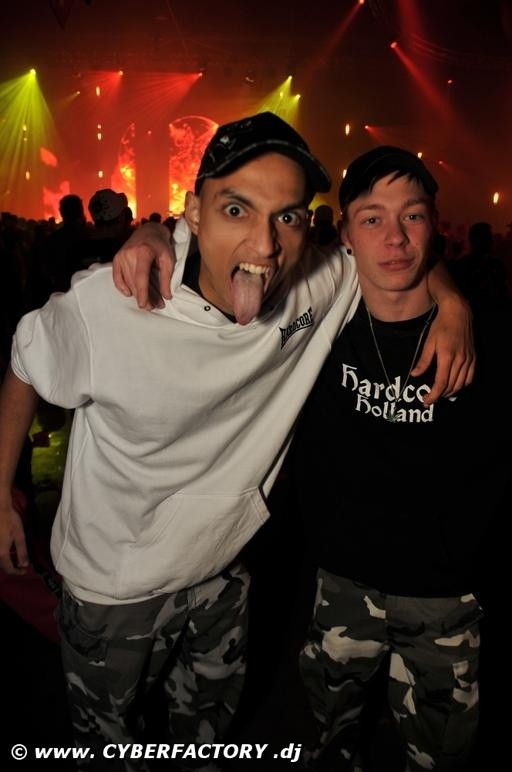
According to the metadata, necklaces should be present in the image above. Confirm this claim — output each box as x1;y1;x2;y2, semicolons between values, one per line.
363;301;438;425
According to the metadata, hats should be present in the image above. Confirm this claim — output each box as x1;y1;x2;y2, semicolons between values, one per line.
193;109;333;192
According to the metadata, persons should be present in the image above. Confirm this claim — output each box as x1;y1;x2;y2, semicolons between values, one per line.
1;188;510;489
1;110;477;771
109;143;511;770
